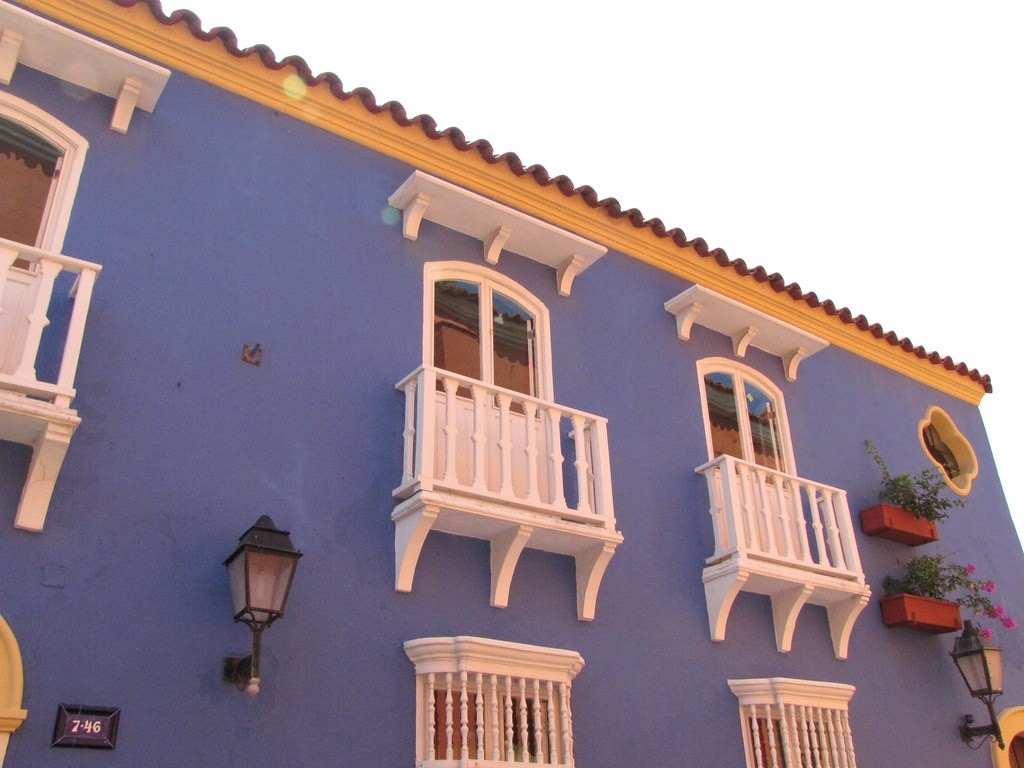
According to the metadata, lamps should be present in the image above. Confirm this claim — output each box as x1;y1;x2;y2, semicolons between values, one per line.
949;621;1007;752
221;515;304;696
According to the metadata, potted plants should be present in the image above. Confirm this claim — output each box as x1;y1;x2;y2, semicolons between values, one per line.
859;439;964;546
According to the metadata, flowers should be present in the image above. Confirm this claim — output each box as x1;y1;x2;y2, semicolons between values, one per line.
883;554;1016;640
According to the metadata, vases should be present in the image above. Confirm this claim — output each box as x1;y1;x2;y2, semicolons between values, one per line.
879;593;963;635
